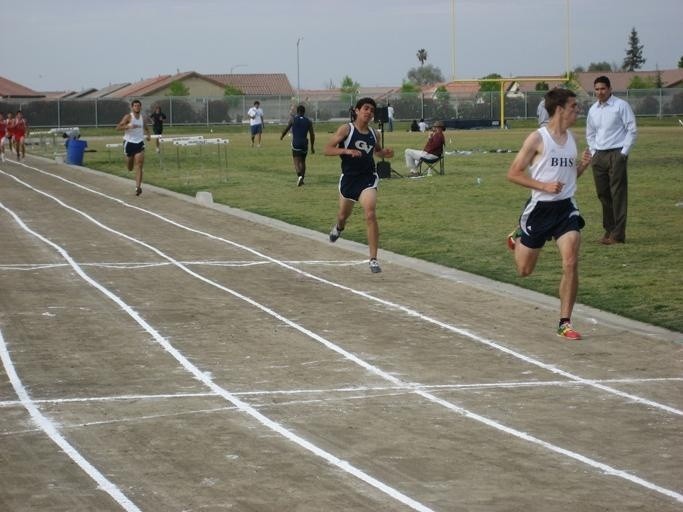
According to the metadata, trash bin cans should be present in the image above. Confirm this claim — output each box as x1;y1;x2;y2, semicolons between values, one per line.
64;139;88;165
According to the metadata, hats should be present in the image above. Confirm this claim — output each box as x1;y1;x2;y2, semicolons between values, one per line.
431;121;444;127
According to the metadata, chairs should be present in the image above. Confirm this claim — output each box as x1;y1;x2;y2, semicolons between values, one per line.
417;143;445;175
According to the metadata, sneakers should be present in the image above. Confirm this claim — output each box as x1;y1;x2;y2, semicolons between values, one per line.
329;223;341;242
507;226;521;251
599;236;624;245
556;320;582;340
134;186;141;197
367;259;381;273
408;172;419;177
297;176;303;187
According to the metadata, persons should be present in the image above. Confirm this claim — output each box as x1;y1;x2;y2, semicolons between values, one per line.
116;100;151;196
387;104;446;179
586;76;638;245
148;104;167;153
349;105;356;123
508;89;592;340
536;98;550;128
0;110;28;164
288;103;297;136
323;97;394;273
280;105;315;187
247;101;265;148
